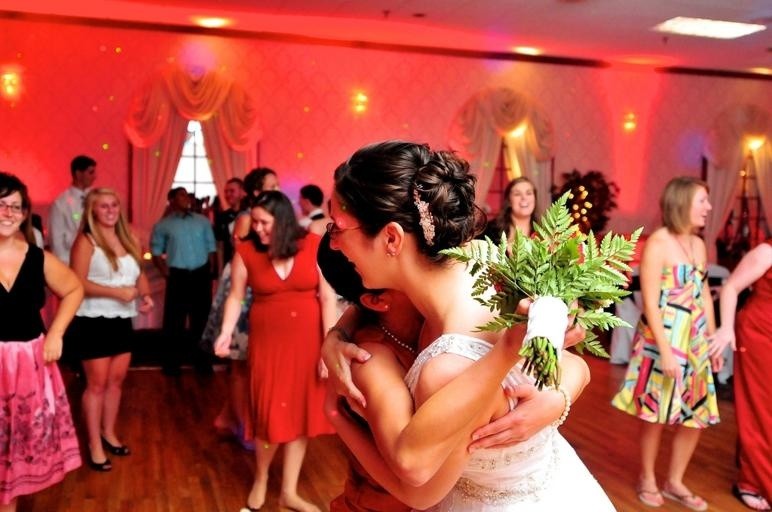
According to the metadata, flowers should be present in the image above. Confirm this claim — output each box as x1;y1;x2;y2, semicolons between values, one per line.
438;188;646;392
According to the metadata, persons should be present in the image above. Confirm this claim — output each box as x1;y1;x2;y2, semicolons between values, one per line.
0;170;84;512
34;156;350;512
320;141;616;512
467;168;771;511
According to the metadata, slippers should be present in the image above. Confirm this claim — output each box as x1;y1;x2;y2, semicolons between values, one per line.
662;489;708;512
635;481;664;507
731;484;771;511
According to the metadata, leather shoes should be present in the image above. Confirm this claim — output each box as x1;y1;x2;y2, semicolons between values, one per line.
85;439;112;471
101;433;131;456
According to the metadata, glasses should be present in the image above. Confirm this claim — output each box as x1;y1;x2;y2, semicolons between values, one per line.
326;219;370;243
0;202;26;216
